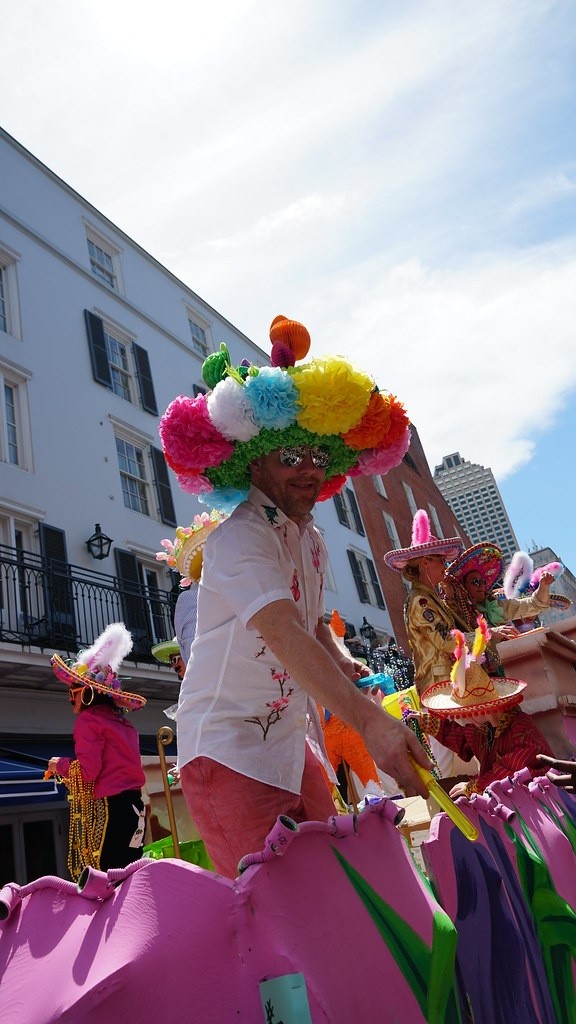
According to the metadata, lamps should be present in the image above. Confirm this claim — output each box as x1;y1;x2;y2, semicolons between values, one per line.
84;523;114;559
360;616;374;639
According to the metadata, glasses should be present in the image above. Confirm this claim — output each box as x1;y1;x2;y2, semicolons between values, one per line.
465;578;486;587
67;687;84;701
270;444;332;469
426;557;447;568
170;655;180;666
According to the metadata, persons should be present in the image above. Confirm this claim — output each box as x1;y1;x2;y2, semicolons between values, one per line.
406;615;555;799
313;448;332;467
384;509;571;708
280;447;304;464
152;637;186;786
49;652;148;889
178;445;436;882
155;511;227;666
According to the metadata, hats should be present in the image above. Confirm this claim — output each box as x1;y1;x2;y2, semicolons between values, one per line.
420;613;528;720
491;551;571;611
49;621;148;714
155;508;231;582
384;508;462;574
151;636;180;664
444;542;507;593
159;316;412;510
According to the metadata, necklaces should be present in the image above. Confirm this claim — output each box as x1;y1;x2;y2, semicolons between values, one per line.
52;761;105;882
402;709;442;781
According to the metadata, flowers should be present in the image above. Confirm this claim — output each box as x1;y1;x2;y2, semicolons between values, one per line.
157;353;414;512
